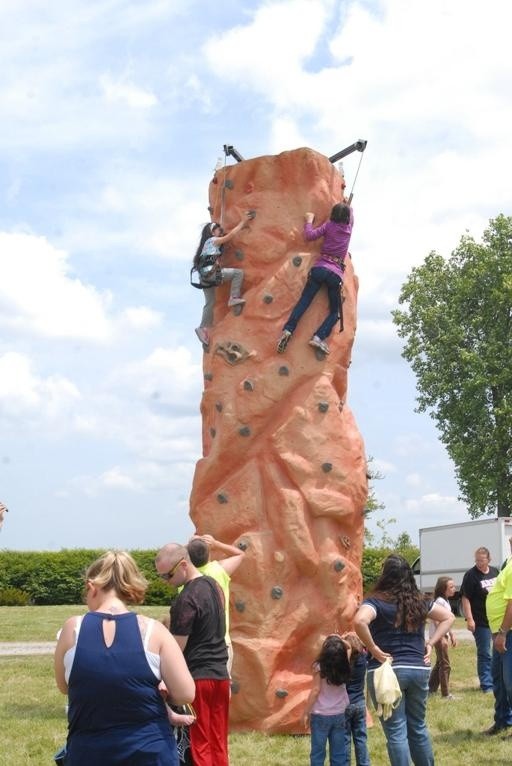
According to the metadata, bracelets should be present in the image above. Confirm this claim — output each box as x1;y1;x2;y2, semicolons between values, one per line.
426;642;435;649
499;627;509;635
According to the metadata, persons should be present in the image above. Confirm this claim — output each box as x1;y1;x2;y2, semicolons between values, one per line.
483;536;512;740
340;633;372;766
354;553;458;766
47;550;196;766
427;576;459;700
152;680;197;728
193;211;254;346
301;633;353;766
276;199;355;354
461;546;501;697
177;533;248;697
150;541;232;766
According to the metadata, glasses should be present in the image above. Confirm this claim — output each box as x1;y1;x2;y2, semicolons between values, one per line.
155;556;185;580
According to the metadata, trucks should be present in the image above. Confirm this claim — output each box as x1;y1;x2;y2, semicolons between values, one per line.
409;517;512;619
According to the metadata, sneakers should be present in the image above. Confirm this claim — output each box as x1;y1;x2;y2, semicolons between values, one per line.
227;296;246;306
277;329;289;354
440;691;458;701
308;336;332;356
480;726;504;736
195;328;211;347
